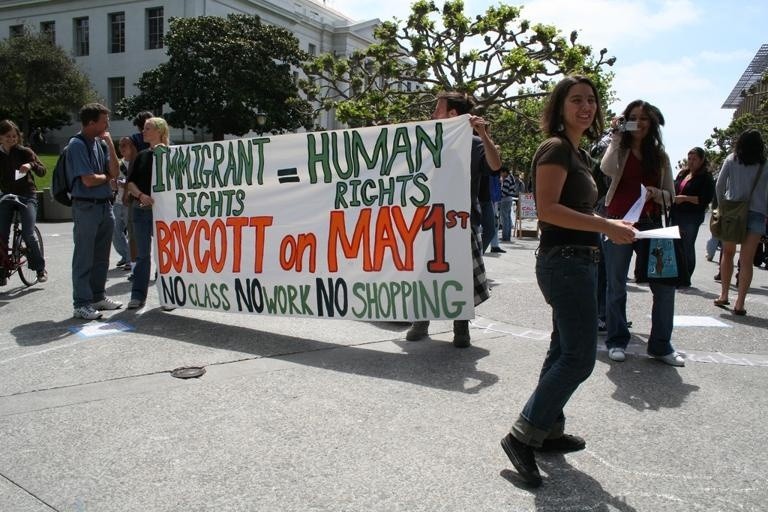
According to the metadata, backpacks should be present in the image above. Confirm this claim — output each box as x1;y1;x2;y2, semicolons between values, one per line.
52;134;88;207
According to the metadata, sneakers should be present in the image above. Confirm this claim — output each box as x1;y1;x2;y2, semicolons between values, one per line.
128;297;146;309
598;318;609;336
95;297;123;310
116;259;126;266
123;264;131;270
609;347;626;362
452;319;471;348
73;303;103;320
703;249;713;261
537;432;586;453
714;272;722;280
37;267;48;282
491;246;507;253
626;321;633;327
405;321;431;342
648;350;685;367
127;274;137;281
161;305;176;311
500;432;543;489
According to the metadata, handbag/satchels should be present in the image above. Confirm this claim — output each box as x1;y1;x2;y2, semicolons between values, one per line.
708;162;766;244
646;191;683;281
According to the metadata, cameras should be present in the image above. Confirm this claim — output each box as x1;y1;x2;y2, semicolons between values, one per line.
615;117;641;131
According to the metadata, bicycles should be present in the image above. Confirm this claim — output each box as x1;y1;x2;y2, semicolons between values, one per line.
0;193;44;287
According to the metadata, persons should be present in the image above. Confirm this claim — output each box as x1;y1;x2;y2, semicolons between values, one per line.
501;73;640;490
671;147;714;288
590;123;632;337
597;100;686;366
0;120;48;288
65;104;124;321
713;128;766;316
512;172;525;199
471;117;497;255
752;238;768;270
714;244;745;287
129;111;152;281
128;118;174;312
110;136;142;272
498;168;516;243
488;143;508;253
405;93;503;349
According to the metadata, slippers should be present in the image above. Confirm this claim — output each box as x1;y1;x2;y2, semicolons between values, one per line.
714;297;730;306
734;306;747;316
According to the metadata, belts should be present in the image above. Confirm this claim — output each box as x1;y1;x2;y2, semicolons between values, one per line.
539;244;601;263
70;195;116;206
